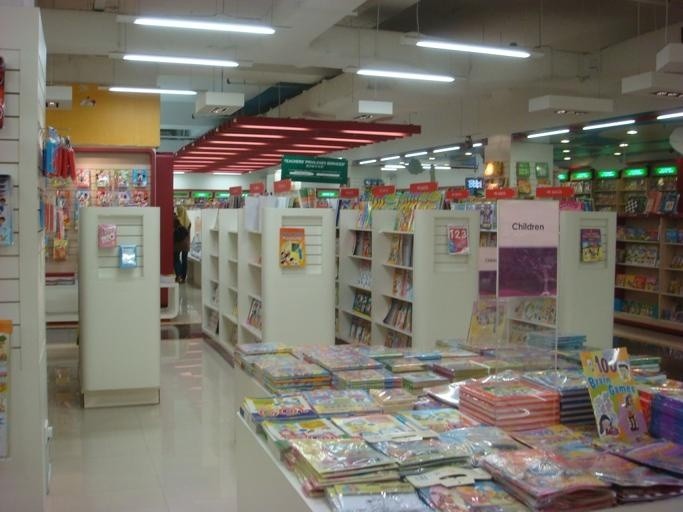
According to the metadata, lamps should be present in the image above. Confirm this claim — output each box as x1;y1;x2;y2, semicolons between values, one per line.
484;160;503;176
108;25;253;68
400;2;548;61
359;79;394;118
343;5;473;84
194;68;245;118
112;2;289;36
97;58;217;94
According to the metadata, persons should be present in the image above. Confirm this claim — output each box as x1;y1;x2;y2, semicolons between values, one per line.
174;205;191;284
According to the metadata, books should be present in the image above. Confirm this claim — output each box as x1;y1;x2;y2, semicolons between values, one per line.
349;200;413;349
614;226;683;323
232;329;683;512
563;178;681;214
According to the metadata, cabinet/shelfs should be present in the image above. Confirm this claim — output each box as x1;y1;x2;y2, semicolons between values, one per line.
202;179;683;356
